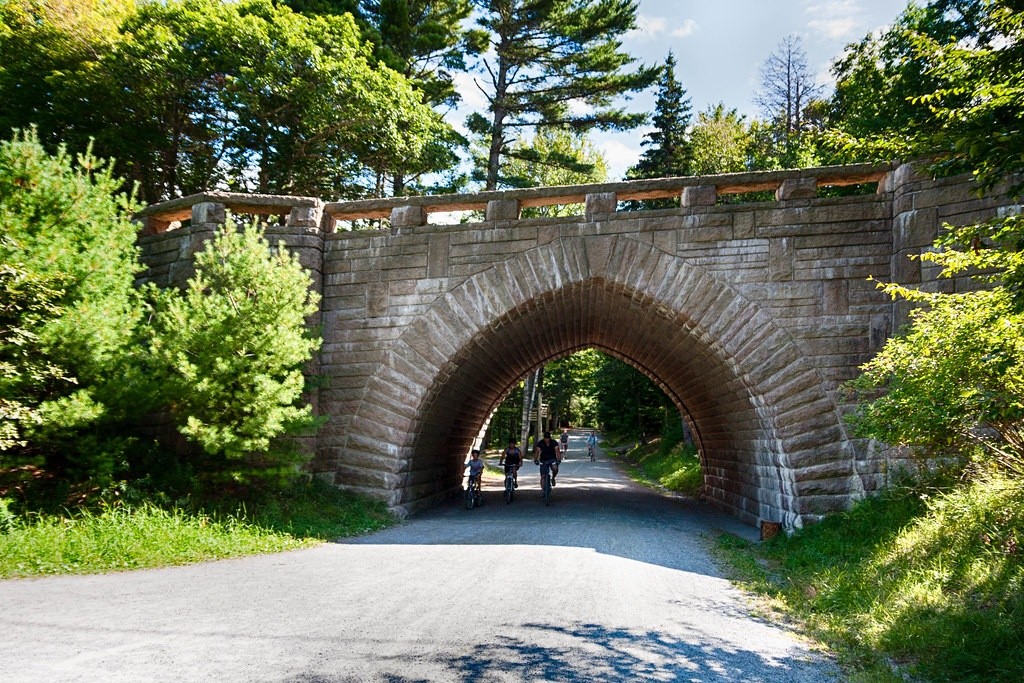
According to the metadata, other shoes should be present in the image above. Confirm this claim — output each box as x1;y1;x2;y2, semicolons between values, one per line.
477;491;481;496
552;479;556;485
513;482;518;488
502;490;508;497
540;493;545;498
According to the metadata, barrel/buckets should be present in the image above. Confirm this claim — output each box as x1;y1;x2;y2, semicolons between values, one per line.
758;520;783;543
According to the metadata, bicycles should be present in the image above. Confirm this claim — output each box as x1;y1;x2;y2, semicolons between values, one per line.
587;444;595;463
461;472;483;509
559;442;568;460
498;463;520;505
534;460;560;506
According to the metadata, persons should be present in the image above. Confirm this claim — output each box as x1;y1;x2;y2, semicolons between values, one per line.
499;438;523;495
587;430;598;461
534;431;562;498
559;430;569;458
460;450;485;500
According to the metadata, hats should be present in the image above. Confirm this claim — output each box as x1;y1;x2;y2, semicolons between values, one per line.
507;438;517;444
544;432;551;438
